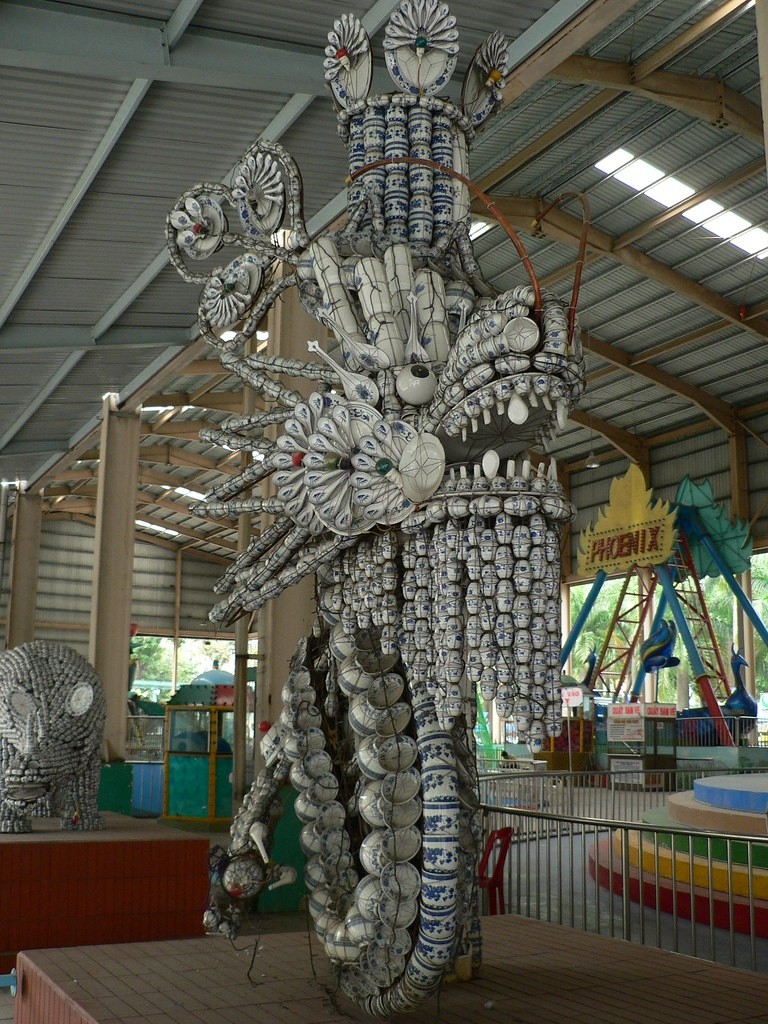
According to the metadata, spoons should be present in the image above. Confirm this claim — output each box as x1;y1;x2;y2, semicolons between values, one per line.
476;31;510;100
272;391;405;535
170;197;209;247
507;388;529;425
204;277;252;327
483;450;500;479
317;307;391;372
323;13;370;80
232;153;285;207
383;0;460;55
306;340;378;408
404;292;430;369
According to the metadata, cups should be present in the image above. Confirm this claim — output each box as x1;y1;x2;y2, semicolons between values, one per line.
299;236;457;422
330;93;477;240
201;752;288;934
313;502;561;755
160;139;365;622
432;284;585;438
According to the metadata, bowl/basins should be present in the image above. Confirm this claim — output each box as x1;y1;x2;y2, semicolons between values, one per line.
281;602;388;964
366;665;470;1015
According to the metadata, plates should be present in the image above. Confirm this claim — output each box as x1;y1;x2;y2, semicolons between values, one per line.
238;176;282;238
289;666;359;917
462;43;501;125
398;433;447;503
333;232;496;297
385;5;458;96
339;969;369;1001
355;628;420;996
454;724;478;938
184;193;225;265
216;253;265;300
328;34;373;104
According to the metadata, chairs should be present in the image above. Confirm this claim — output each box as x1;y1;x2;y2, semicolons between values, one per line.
478;826;515;917
